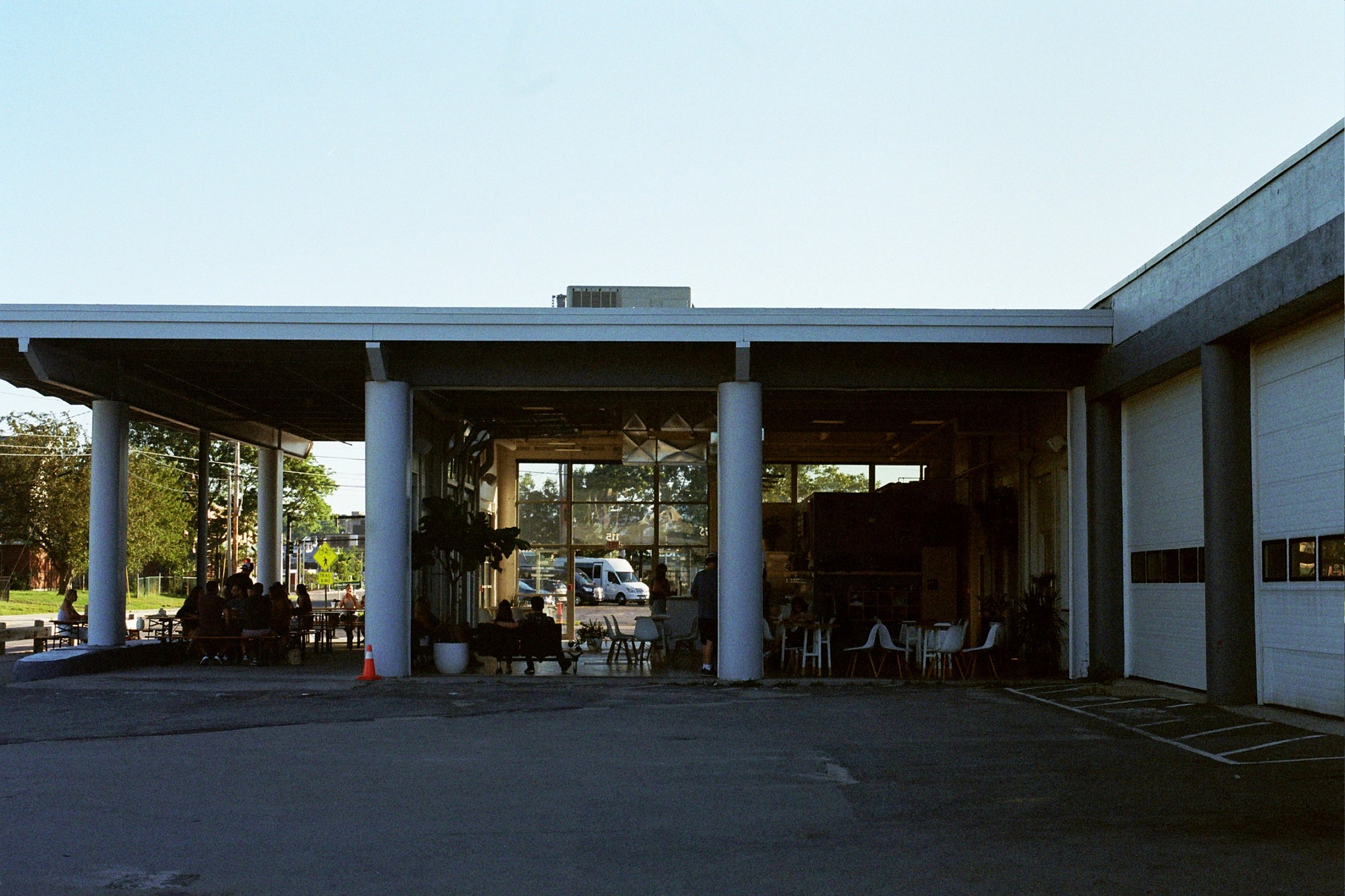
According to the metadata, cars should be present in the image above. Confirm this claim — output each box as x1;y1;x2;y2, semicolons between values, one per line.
518;577;567;606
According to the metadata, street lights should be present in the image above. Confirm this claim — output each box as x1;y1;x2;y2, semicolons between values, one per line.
294;543;313;592
327;543;341;592
302;534;318;585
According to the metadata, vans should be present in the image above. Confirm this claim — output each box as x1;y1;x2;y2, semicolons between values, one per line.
553;556;650;606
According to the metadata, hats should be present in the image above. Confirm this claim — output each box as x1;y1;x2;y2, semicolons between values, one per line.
704;551;718;561
239;562;255;572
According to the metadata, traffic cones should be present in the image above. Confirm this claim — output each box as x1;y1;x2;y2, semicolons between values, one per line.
355;644;382;680
554;600;564;623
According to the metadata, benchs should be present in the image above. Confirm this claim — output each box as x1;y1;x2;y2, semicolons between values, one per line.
474;620;578;676
35;620;369;662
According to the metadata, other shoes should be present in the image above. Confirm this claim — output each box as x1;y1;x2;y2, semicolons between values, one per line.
558;661;572;672
495;667;503;675
524;666;535;674
701;668;718;677
253;656;264;666
505;668;512;674
197;652;210;665
214;652;225;666
242;654;249;667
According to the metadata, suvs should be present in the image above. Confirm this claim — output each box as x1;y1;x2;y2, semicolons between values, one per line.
518;565;605;606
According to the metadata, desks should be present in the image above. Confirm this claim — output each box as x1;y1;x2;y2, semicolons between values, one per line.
775;621;838;679
145;607;365;655
635;614;672;663
902;619;950;680
48;620;87;648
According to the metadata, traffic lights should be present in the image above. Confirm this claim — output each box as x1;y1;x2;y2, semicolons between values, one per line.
243;558;250;564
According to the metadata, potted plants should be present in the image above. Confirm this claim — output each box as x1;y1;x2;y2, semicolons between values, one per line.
575;619;609;652
411;496;531;676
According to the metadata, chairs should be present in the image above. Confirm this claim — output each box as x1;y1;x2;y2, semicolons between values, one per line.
605;599;697;667
410;617;436;669
847;615;1000;685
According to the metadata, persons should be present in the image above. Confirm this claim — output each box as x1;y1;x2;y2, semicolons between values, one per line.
330;585;365;649
58;589;88;643
178;561;313;665
488;596;572;675
690;551;719;676
783;609;838;667
648;563;678;655
763;567;776;673
